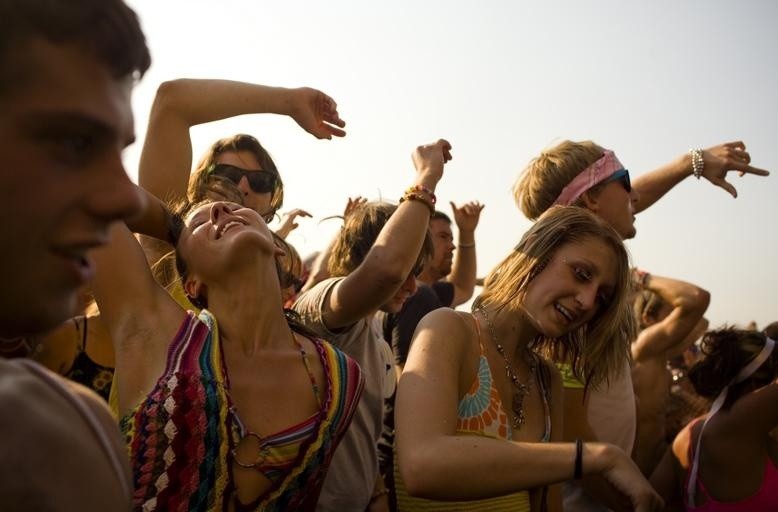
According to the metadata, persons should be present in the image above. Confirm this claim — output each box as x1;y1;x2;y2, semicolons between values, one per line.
2;1;150;509
63;80;363;510
292;138;778;511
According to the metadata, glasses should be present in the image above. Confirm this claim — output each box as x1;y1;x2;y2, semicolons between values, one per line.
598;170;632;193
208;164;277;194
